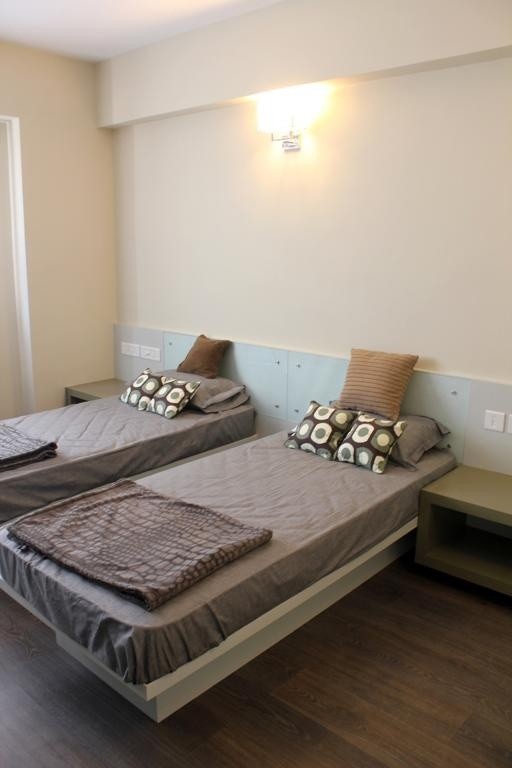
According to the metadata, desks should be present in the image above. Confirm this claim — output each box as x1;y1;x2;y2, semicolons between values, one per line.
415;465;512;598
65;378;131;406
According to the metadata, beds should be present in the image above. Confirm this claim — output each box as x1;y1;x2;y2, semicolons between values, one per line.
0;396;453;723
0;430;454;723
1;397;258;523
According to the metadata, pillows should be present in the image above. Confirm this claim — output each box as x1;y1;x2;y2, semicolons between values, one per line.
336;347;420;420
331;412;408;474
119;333;249;418
284;400;358;461
286;400;450;471
